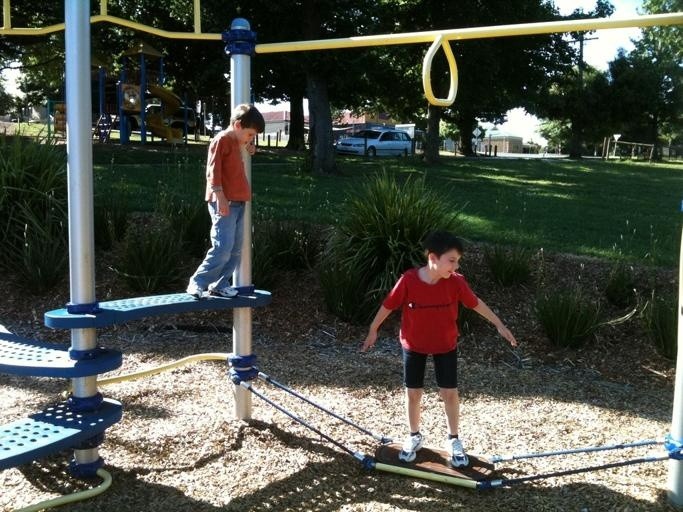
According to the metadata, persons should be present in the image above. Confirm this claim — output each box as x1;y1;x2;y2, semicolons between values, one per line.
186;104;266;300
363;231;518;469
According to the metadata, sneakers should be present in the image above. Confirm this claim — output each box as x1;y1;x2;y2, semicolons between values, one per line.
443;434;470;469
207;281;239;298
186;276;202;299
398;433;423;462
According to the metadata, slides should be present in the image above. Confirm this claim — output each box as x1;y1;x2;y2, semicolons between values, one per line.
147;89;183;145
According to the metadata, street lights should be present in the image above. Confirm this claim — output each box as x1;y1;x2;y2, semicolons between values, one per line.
571;34;587;161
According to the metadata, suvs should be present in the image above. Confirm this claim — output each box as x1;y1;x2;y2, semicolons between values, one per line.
113;103;197;140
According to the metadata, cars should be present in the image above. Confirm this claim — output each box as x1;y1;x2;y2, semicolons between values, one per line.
336;129;413;160
